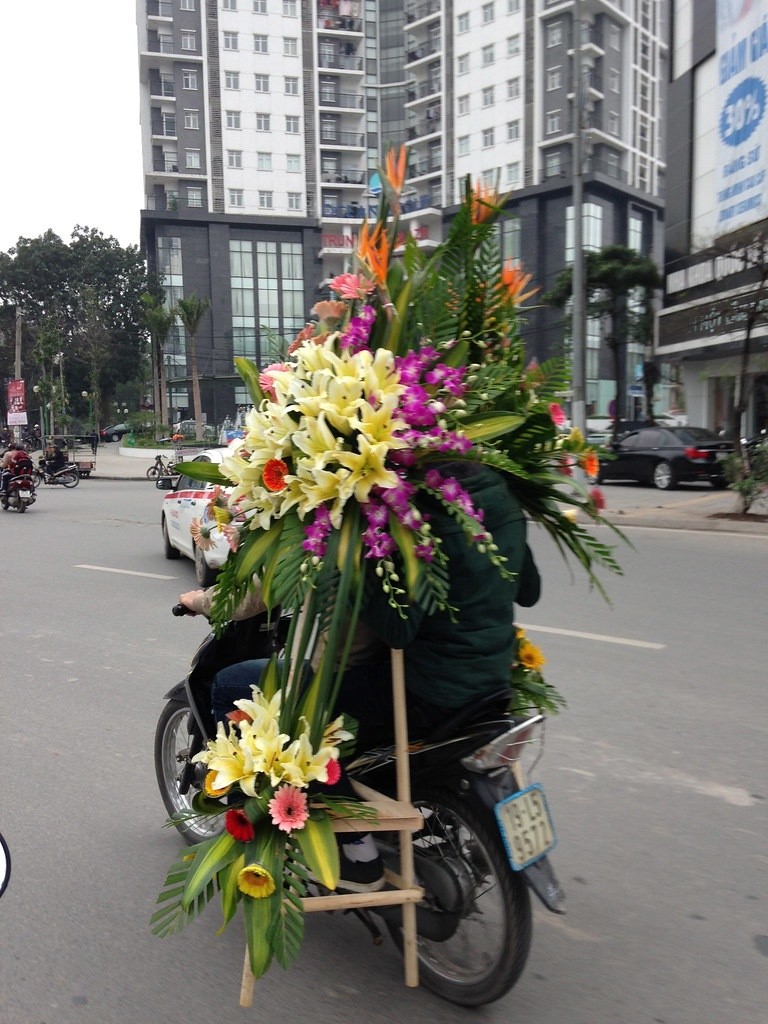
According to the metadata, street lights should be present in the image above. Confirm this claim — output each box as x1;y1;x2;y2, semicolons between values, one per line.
111;401;128;421
80;390;98;431
33;385;57;445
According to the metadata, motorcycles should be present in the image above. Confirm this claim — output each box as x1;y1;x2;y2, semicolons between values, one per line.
151;597;569;1007
29;462;79;488
1;423;43;453
0;458;37;513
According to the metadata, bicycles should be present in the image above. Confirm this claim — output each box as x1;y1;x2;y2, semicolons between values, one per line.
147;455;178;482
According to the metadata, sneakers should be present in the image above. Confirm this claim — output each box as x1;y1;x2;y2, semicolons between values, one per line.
292;848;387;894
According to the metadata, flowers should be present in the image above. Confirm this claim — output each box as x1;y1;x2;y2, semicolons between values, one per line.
149;128;632;979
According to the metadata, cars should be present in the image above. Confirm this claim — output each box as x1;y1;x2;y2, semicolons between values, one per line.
172;418;212;436
154;448;271;591
588;426;738;490
100;422;143;442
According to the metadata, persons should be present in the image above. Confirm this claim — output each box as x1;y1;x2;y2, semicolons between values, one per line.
0;413;84;513
89;428;100;456
317;422;546;895
169;406;329;857
